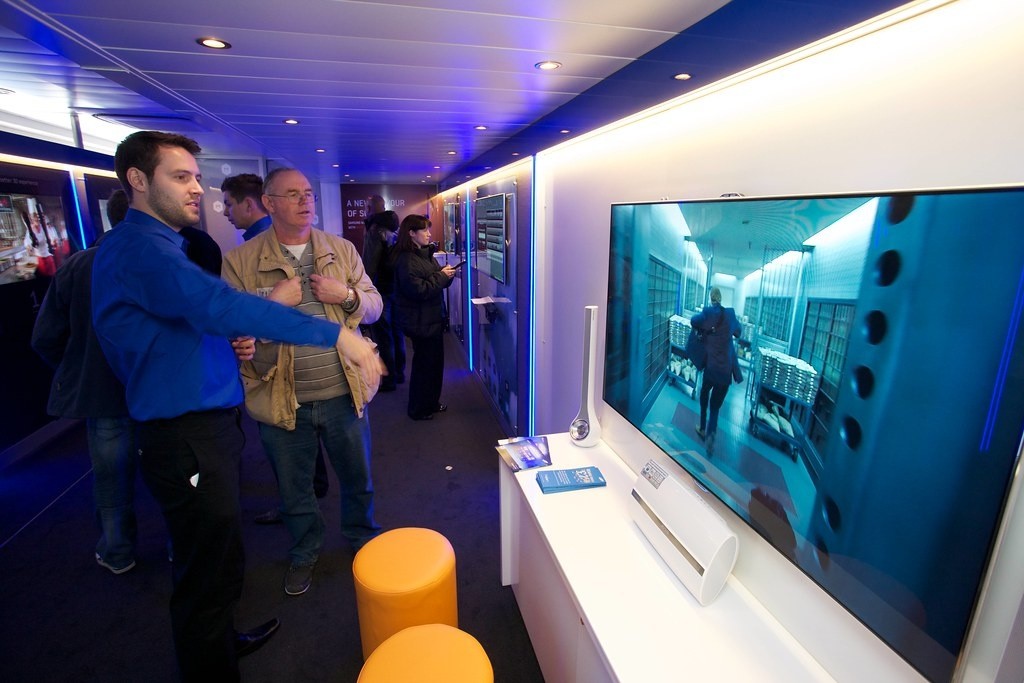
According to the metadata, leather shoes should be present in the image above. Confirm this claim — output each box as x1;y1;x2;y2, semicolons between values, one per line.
238;617;280;657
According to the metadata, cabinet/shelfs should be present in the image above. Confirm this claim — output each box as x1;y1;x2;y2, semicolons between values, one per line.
750;371;813;462
667;342;699;400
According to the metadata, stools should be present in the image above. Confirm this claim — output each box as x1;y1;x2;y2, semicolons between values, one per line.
357;624;494;683
353;527;458;664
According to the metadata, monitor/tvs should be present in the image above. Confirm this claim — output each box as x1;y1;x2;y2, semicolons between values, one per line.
444;193;506;285
602;185;1023;683
0;160;86;296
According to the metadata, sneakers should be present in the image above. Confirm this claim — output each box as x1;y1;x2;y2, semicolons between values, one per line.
95;552;136;575
168;558;172;561
284;561;315;594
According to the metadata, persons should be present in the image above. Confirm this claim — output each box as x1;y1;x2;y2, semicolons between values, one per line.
363;194;407;391
26;211;59;276
33;189;174;574
91;132;385;683
382;210;405;245
390;215;456;420
691;287;744;457
221;173;329;523
47;222;60;250
178;224;221;277
221;169;386;594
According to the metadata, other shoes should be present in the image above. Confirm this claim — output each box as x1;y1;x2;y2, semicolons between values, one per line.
437;400;447;412
254;507;283;524
378;383;397;392
696;425;706;439
421;413;433;421
397;376;406;384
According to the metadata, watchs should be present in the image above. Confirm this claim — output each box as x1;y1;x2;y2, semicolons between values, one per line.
341;289;354;308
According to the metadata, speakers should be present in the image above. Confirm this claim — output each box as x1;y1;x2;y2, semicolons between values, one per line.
568;306;603;448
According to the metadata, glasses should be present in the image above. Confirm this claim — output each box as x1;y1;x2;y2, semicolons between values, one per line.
268;191;314;205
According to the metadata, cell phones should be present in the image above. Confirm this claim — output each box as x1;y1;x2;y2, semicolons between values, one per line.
451;260;467;270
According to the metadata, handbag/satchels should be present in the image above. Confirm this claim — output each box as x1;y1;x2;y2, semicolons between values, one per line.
686;332;709;371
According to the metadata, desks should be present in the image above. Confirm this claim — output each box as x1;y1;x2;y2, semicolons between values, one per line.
497;433;838;683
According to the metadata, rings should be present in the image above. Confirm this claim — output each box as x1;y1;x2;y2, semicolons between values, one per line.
450;272;452;275
